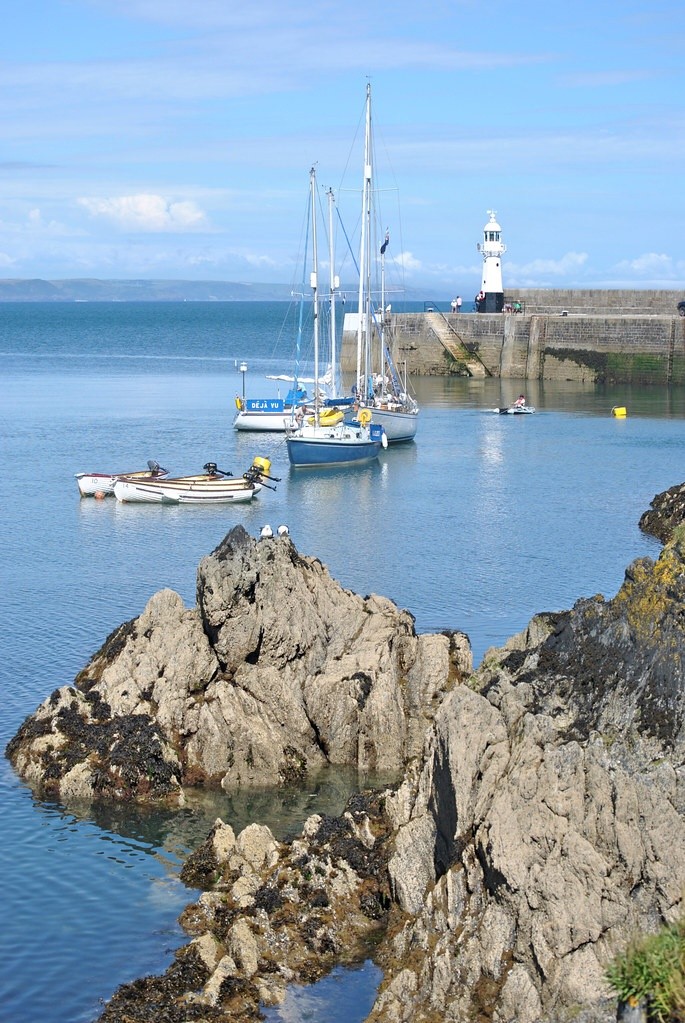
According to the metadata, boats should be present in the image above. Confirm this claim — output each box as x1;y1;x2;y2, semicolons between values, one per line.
493;406;535;414
74;457;281;504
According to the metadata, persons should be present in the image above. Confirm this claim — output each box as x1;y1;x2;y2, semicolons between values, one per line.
505;300;522;315
512;394;525;408
297;383;307;401
295;405;314;430
451;296;462;313
475;294;483;308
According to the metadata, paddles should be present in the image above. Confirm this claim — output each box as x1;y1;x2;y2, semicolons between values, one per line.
518;403;535;414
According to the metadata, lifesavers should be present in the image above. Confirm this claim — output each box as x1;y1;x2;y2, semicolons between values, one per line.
479;290;485;300
235;398;241;409
358;408;372;422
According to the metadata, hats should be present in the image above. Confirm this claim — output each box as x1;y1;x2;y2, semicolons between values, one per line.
520;395;524;397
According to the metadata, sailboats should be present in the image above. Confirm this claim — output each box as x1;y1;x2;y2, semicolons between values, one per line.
232;83;418;469
